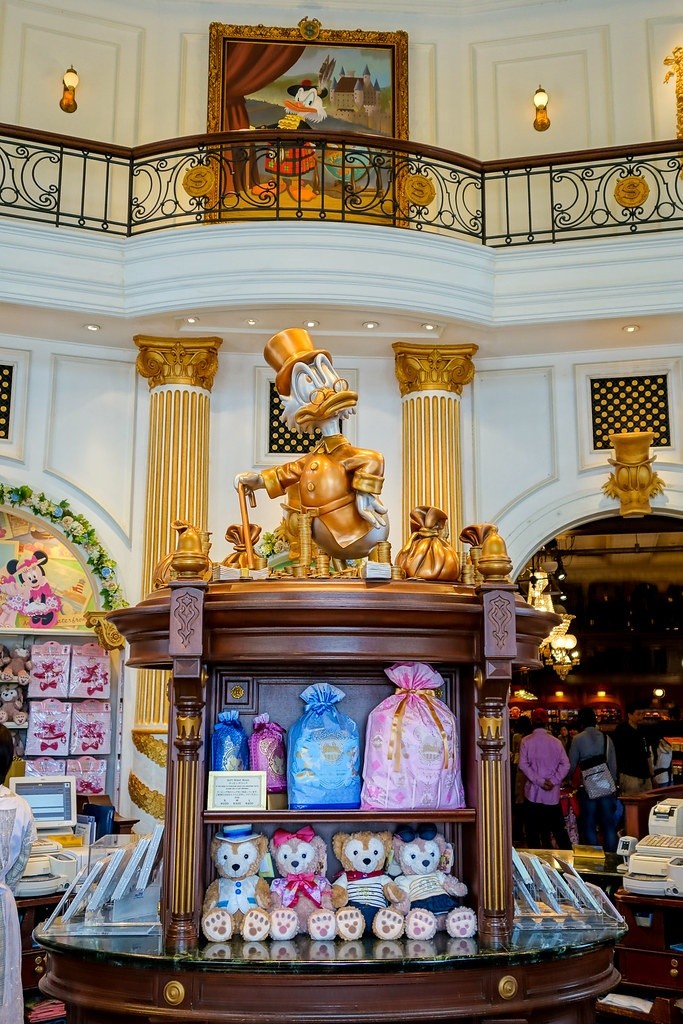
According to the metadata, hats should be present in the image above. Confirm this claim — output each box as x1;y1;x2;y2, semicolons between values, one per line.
578;707;597;727
532;708;548;724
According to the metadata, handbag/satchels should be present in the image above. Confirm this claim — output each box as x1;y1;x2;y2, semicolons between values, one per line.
582;762;616;800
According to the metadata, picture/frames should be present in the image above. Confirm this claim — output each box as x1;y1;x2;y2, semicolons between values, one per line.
202;18;412;232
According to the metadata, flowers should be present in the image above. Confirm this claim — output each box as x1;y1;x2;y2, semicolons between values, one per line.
0;483;130;610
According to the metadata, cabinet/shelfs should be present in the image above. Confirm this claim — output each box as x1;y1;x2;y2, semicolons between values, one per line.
0;579;682;1024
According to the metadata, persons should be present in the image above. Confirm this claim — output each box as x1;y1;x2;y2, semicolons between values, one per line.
510;706;683;854
0;724;38;1024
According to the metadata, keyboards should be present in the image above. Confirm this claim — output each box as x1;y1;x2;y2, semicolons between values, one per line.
635;834;683;857
30;839;58;851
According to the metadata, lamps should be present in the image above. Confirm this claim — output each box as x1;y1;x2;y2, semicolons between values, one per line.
533;84;550;132
59;65;80;113
552;557;567;579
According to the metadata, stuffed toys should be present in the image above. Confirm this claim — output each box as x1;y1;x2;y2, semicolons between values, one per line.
330;830;404;940
0;643;34;759
201;824;272;943
392;824;477;941
266;824;336;940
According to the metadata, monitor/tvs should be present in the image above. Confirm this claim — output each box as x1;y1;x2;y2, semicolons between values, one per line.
9;776;76;836
